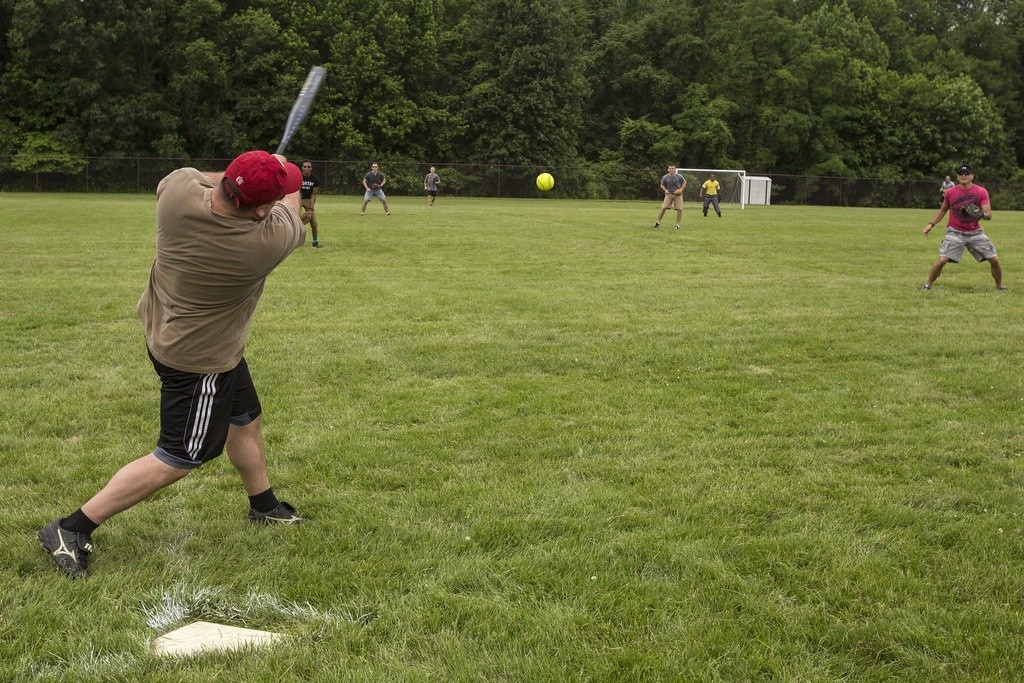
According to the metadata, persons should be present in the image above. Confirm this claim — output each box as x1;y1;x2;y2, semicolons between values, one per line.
359;161;390;215
700;173;721;217
939;175;955;206
921;164;1006;292
299;160;320;246
424;167;440;206
653;163;687;229
39;150;306;576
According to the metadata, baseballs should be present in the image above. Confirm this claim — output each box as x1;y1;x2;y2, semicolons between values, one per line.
536;172;555;192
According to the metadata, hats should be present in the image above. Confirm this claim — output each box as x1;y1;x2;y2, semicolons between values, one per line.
957;165;974;174
223;150;303;209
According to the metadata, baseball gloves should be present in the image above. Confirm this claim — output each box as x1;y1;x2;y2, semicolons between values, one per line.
958;203;984;220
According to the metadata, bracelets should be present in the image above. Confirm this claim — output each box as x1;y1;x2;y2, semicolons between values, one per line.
929;222;934;227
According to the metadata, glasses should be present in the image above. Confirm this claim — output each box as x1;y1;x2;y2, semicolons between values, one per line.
958;171;971;175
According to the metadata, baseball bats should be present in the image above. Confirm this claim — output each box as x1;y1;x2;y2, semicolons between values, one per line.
275;65;327;156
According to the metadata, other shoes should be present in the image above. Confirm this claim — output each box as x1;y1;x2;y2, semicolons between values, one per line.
654;222;660;227
675;225;679;229
923;284;931;291
313;241;318;246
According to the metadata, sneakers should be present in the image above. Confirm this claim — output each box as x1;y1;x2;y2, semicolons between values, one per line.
250;501;300;524
38;516;94;581
